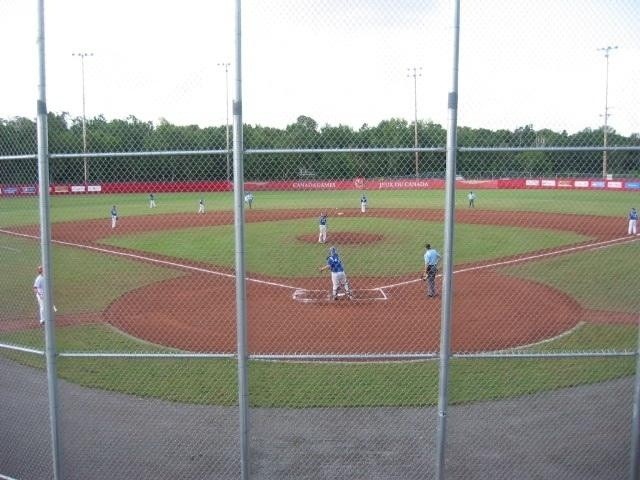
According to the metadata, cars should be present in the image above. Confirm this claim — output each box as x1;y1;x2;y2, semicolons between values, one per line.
456;175;467;182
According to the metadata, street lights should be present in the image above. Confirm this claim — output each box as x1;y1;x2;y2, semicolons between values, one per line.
407;65;424;179
597;44;619;181
70;50;95;184
217;62;232;183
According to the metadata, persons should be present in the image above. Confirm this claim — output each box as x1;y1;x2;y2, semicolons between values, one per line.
360;194;367;212
318;246;352;300
422;242;440;297
628;208;638;236
318;212;328;244
110;205;118;228
466;191;477;207
149;194;157;209
245;192;253;208
32;265;46;325
197;197;205;214
